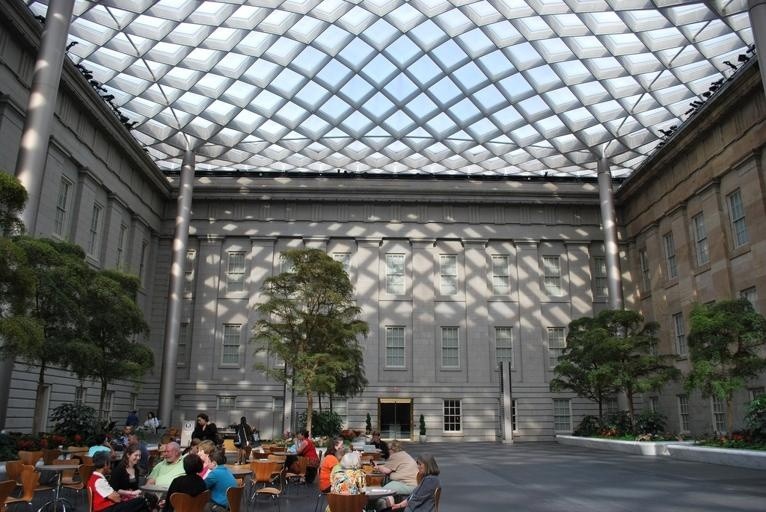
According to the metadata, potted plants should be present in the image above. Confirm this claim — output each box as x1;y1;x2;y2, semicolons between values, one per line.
364;413;374;443
419;414;427;444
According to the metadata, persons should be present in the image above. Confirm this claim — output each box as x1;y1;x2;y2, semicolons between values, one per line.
124;409;138;428
88;428;153;511
191;414;219;445
233;416;255;465
143;411;159;436
288;427;440;511
147;427;238;511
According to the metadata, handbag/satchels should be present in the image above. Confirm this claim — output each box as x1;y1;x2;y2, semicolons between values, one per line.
247;441;252;448
234;430;241;446
